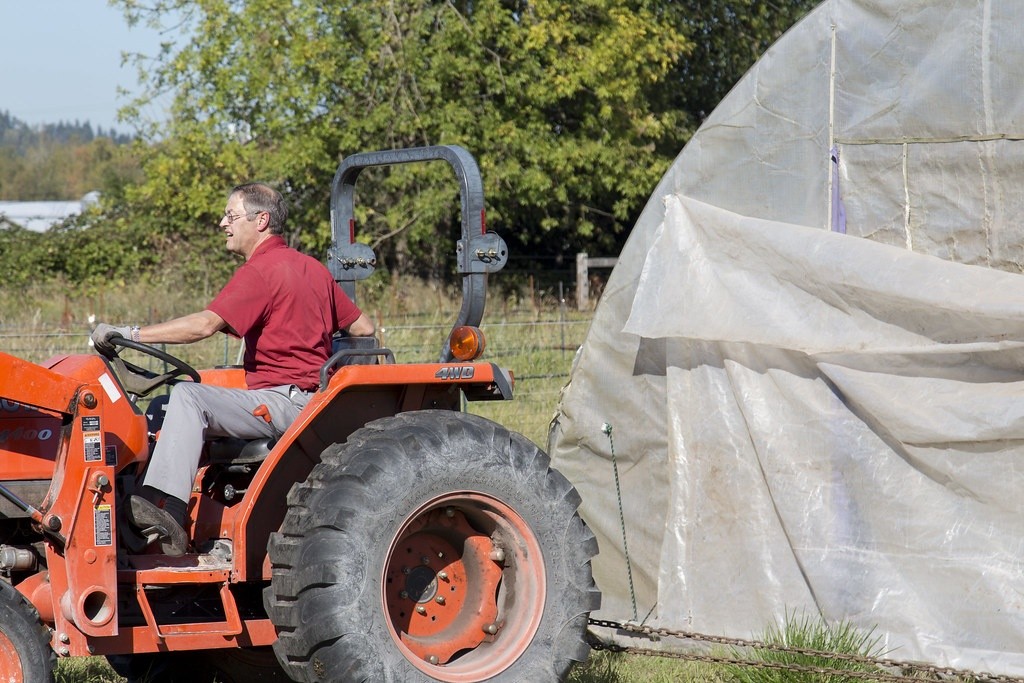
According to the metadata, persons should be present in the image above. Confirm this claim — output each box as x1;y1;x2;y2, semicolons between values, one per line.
88;182;377;557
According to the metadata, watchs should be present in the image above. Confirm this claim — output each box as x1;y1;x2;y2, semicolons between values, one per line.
130;325;140;343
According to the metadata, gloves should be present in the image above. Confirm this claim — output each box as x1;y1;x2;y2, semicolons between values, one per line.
91;322;133;361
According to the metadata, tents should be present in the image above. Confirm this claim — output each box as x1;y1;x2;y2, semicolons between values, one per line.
544;1;1023;679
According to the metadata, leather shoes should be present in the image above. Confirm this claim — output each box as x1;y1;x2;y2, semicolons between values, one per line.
116;472;189;558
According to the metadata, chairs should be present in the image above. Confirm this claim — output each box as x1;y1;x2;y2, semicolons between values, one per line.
206;337;380;474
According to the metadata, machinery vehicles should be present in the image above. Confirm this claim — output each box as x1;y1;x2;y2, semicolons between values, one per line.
0;146;602;683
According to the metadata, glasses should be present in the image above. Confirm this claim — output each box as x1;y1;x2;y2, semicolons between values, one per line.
224;211;263;221
